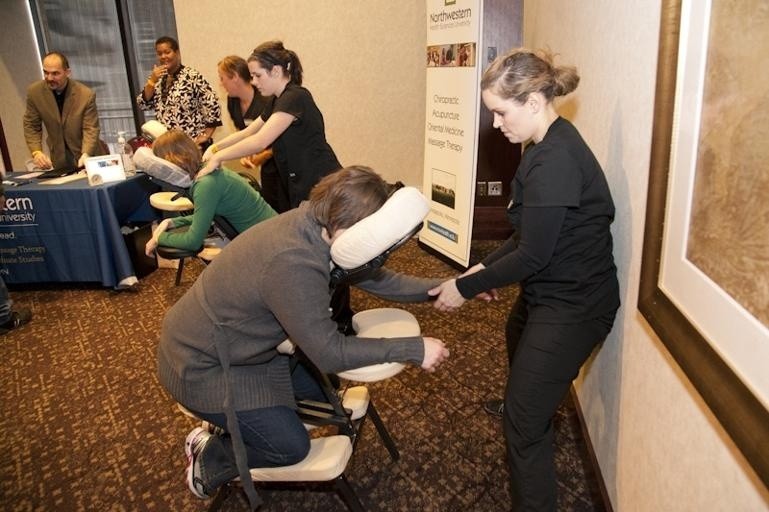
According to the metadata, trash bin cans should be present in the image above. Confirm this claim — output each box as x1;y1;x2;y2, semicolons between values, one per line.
120;220;158;280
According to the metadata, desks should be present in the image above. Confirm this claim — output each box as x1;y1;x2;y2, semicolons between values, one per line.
0;172;164;290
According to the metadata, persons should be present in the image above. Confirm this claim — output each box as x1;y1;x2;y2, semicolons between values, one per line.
23;52;107;172
136;36;224;157
1;276;32;335
194;40;354;337
154;164;500;500
217;56;288;216
144;129;280;259
425;48;620;510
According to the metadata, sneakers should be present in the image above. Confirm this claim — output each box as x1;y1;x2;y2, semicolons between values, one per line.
201;419;227;437
182;424;227;502
0;306;33;335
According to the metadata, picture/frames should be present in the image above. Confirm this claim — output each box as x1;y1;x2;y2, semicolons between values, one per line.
638;0;769;507
